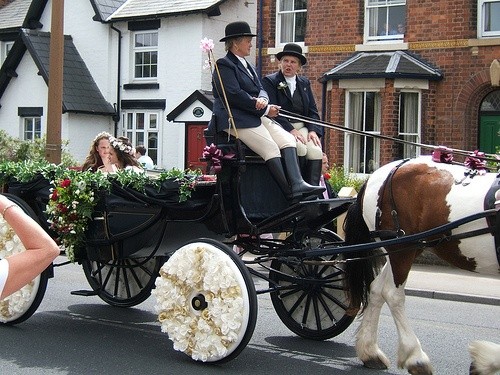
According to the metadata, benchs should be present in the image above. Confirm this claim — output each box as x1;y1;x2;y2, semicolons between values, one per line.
203;114;291;232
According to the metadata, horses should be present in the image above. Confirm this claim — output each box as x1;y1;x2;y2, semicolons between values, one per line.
342;155;500;375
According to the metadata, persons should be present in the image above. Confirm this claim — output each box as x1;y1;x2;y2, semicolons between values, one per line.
232;232;295;255
134;145;154;170
318;152;336;234
106;136;145;173
211;21;327;205
379;23;407;35
81;131;115;172
261;43;322;201
0;193;61;300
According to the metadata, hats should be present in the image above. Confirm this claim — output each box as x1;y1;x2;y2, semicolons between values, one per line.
219;21;258;43
275;43;307;65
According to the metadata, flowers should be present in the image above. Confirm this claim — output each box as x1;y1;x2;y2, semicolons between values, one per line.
0;158;245;362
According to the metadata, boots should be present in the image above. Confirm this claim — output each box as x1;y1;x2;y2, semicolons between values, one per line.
280;147;327;199
297;155;324;199
265;157;319;207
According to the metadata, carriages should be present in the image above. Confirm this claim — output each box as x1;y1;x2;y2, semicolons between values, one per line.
0;146;500;375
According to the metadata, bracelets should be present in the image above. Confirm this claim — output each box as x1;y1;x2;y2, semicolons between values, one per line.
2;204;19;218
